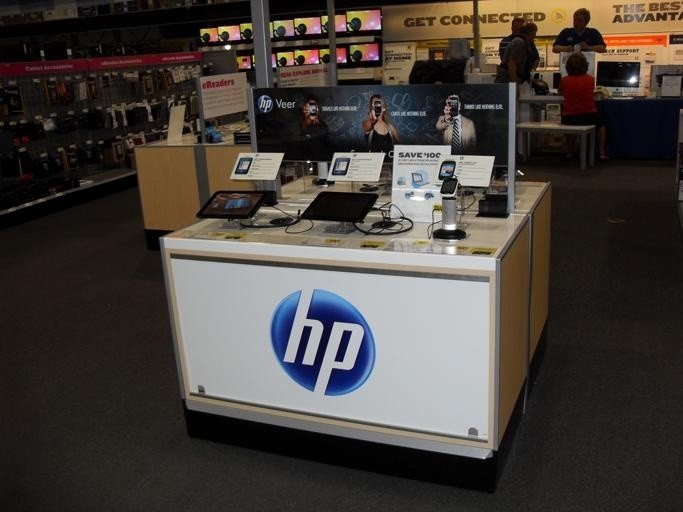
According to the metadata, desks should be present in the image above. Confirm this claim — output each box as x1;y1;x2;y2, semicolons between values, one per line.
134;120;257;252
518;94;682;156
279;172;550;385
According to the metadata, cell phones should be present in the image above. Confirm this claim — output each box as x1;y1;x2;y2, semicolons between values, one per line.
332;157;351;176
308;101;318;117
373;100;382;118
440;177;460;198
234;156;254;175
448;97;459;117
438;160;457;181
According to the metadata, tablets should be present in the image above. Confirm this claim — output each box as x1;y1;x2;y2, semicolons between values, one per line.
412;172;423;183
197;190;268;218
301;191;379;221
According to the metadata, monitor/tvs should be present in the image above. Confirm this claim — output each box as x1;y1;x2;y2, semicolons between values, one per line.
595;60;642;93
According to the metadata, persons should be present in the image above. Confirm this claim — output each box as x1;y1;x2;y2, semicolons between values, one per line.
436;93;477;156
495;22;537;176
558;53;609;160
499;18;540;72
363;94;399;161
553;8;607;53
300;94;329;161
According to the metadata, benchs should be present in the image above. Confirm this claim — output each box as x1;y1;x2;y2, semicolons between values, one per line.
515;120;595;172
